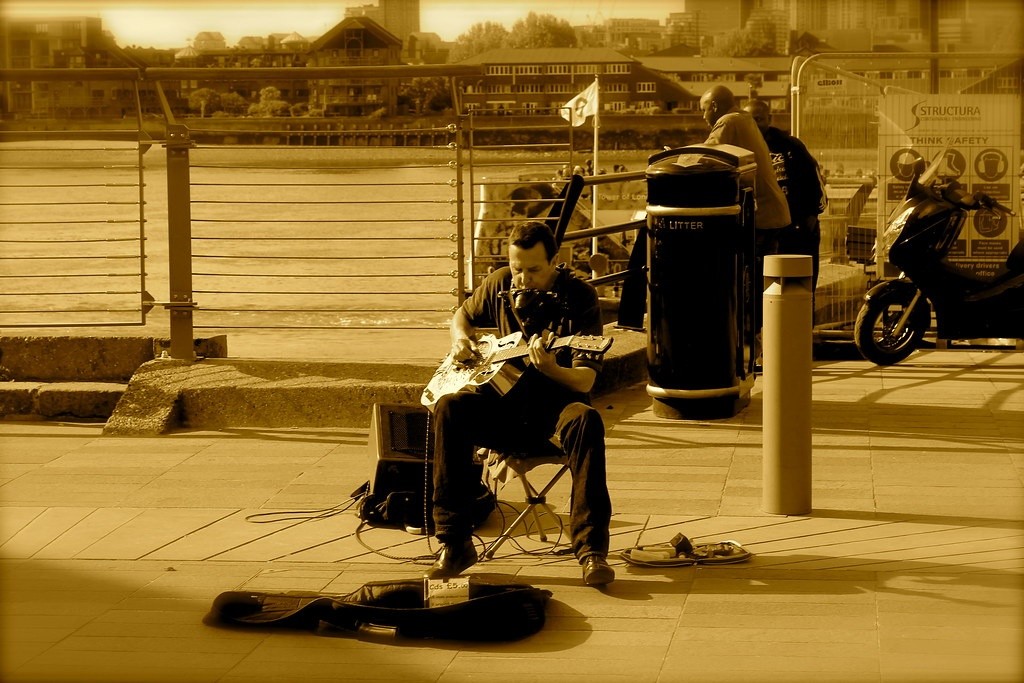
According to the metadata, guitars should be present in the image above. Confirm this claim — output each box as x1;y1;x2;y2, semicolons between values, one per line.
420;331;614;413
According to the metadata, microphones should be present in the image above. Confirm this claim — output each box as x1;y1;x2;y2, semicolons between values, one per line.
514;286;534;309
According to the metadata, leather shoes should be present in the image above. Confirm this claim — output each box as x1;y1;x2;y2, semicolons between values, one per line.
423;539;478;578
582;555;615;584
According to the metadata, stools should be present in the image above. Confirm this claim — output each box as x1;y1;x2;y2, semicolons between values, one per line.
482;447;576;559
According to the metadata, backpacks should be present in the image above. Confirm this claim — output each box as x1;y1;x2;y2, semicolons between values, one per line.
201;578;554;644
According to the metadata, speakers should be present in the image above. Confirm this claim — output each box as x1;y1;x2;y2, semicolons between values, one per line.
364;402;435;523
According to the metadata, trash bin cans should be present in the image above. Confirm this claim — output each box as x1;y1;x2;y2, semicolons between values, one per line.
644;141;758;422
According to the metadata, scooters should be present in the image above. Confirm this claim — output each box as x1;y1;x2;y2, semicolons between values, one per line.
852;142;1024;369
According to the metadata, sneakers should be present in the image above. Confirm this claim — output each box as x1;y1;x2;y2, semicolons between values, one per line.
753;362;763;375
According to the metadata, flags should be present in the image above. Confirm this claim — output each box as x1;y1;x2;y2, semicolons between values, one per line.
560;81;597;127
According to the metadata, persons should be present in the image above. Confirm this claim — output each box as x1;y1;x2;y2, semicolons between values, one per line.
425;221;618;586
739;99;829;332
664;84;793;376
